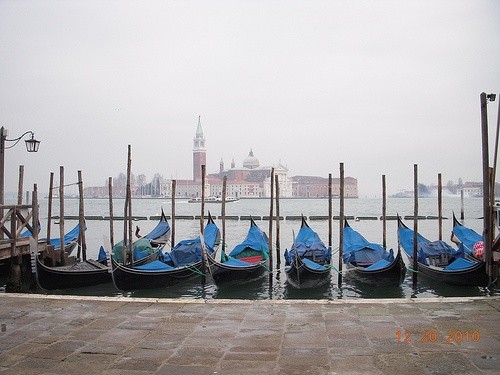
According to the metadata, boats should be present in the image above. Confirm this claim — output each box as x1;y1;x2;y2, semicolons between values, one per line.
109;210;221;294
37;215;87;263
188;195;240;203
396;212;487;286
451;211;500;281
36;206;172;292
205;214;272;290
19;218;42;239
342;216;408;287
284;214;332;290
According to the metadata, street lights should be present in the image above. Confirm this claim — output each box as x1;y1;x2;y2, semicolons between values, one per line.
480;92;496;276
0;127;40;240
